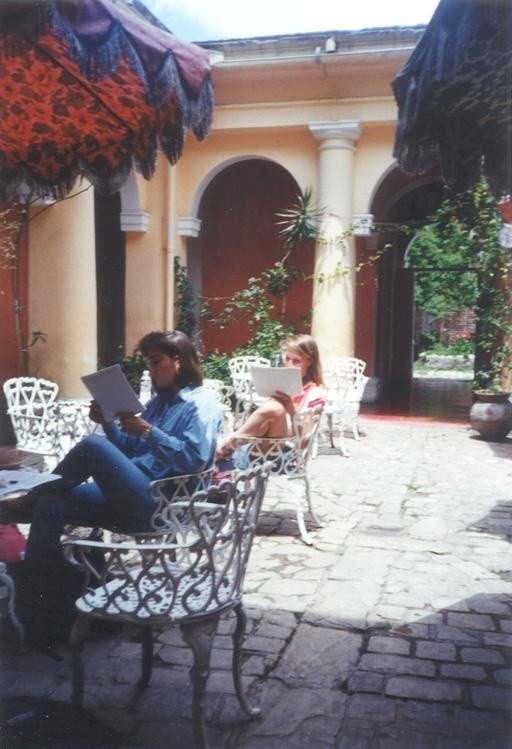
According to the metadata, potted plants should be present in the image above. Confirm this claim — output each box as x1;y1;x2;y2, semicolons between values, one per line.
429;154;512;441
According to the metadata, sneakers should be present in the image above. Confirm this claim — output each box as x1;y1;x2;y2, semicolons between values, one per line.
0;495;36;524
214;445;234;462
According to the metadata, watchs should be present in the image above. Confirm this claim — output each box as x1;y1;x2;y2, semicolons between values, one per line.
142;424;153;439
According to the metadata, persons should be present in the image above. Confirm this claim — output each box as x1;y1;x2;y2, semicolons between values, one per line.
0;328;226;580
0;383;19;467
208;332;328;505
